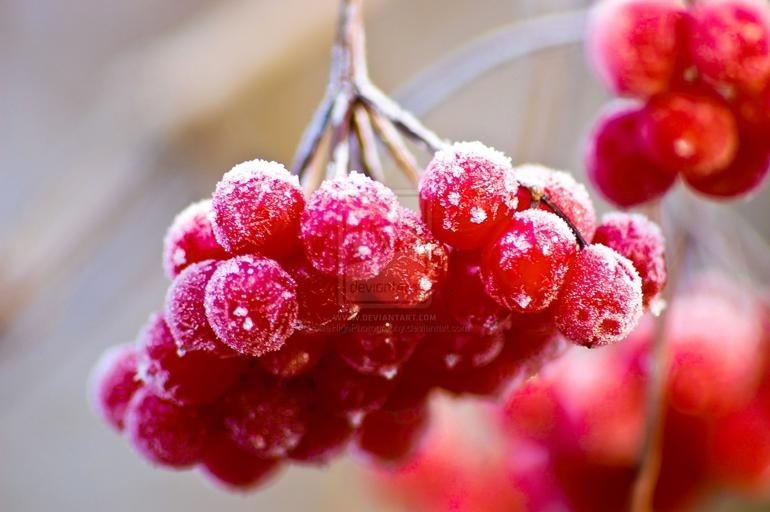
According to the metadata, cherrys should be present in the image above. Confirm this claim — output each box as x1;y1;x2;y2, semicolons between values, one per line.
91;1;770;491
